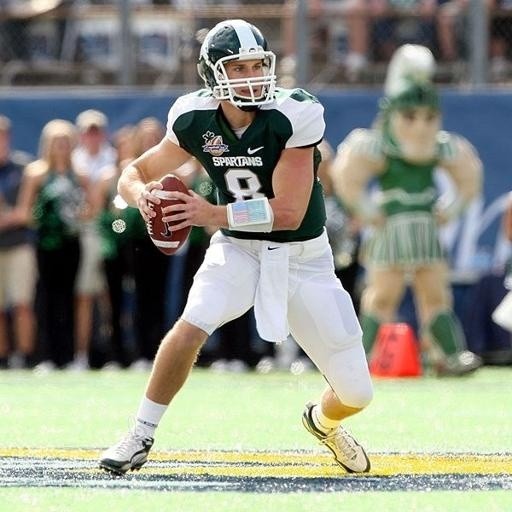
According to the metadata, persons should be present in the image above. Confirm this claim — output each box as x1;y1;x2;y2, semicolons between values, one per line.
1;1;512;89
94;18;381;475
329;44;485;377
2;110;512;376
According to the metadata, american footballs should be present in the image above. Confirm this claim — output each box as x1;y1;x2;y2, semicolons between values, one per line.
146;176;191;254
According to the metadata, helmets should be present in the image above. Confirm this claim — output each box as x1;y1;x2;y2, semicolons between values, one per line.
195;17;279;107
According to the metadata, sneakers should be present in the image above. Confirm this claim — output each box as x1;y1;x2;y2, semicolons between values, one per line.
433;350;484;379
300;400;372;474
96;433;156;475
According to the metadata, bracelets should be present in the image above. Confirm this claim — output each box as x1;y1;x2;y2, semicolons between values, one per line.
224;197;274;237
